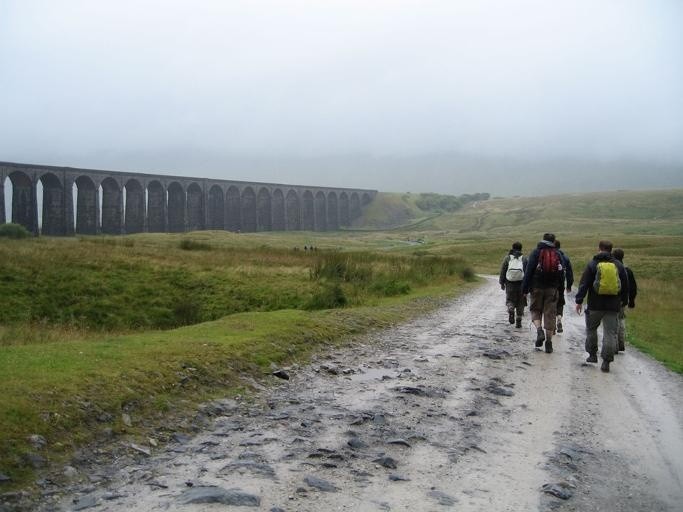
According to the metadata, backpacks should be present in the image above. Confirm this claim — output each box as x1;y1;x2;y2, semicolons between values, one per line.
532;247;564;284
506;254;525;281
593;256;622;295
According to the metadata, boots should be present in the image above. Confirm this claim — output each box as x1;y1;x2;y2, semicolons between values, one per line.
618;339;625;351
545;340;553;353
557;319;563;333
586;352;597;362
535;330;545;346
601;359;609;372
508;307;523;328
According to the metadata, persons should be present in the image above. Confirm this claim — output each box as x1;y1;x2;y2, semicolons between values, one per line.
519;232;563;354
574;239;628;374
553;239;573;335
611;248;636;355
498;242;528;328
